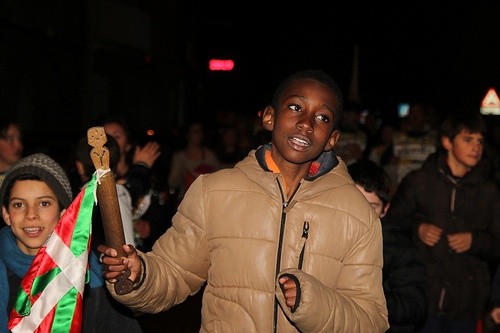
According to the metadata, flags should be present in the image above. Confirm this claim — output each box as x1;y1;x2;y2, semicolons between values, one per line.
7;176;99;333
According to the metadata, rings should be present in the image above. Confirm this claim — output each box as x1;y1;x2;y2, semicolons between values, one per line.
100;254;104;262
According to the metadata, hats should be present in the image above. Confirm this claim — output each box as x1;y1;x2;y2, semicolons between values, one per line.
0;153;73;207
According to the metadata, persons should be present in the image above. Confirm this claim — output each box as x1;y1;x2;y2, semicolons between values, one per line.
332;96;440;191
98;71;389;333
387;111;500;333
485;118;500;186
74;113;271;253
0;153;142;333
0;120;23;182
346;161;429;333
